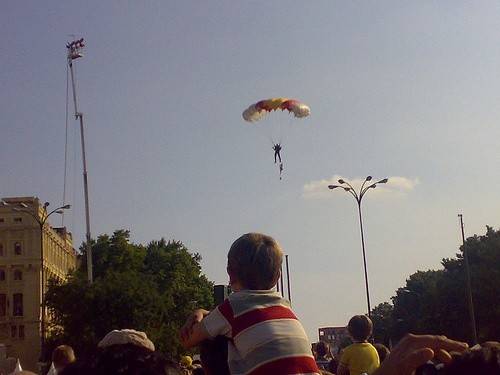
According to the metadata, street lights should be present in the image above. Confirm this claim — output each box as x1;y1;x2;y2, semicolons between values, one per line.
326;175;389;343
1;199;71;364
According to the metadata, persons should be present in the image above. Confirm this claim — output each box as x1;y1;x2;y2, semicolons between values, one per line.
178;232;321;375
0;315;500;375
272;143;282;163
